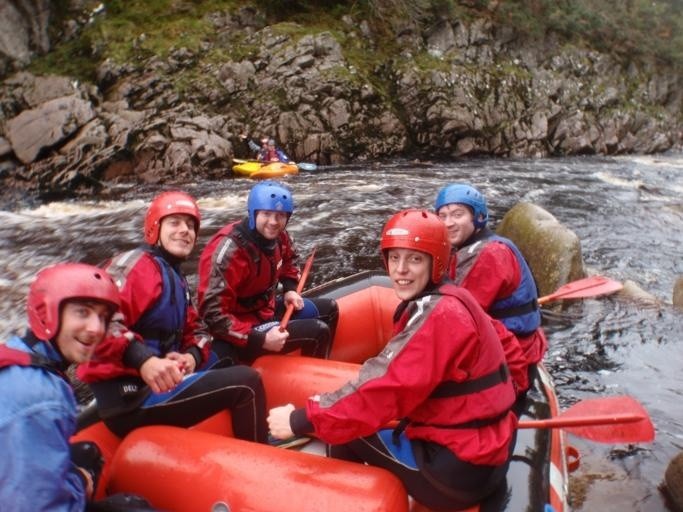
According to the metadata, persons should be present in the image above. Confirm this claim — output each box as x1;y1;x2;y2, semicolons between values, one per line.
256;137;288;162
192;181;339;359
265;208;516;510
437;182;550;411
75;190;267;445
0;262;151;512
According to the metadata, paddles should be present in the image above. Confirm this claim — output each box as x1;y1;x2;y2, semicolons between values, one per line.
383;398;654;444
232;158;319;171
537;275;622;306
279;247;318;333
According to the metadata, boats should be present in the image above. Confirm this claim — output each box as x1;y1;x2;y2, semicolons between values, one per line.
232;158;301;178
65;269;571;512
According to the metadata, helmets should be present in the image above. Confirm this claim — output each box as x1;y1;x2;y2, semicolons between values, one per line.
248;180;293;230
144;192;201;246
379;211;451;285
28;263;121;344
435;184;488;227
